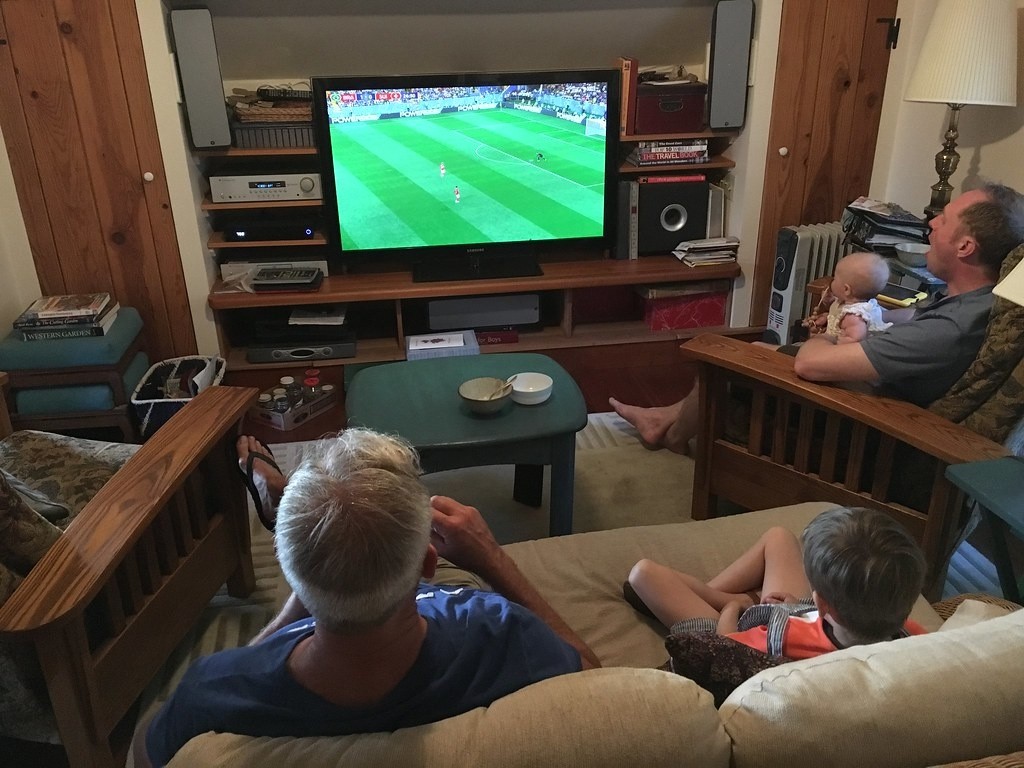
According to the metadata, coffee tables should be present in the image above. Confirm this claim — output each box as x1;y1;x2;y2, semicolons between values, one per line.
343;352;589;538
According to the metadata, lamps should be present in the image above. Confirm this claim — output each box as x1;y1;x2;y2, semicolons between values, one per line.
903;0;1018;245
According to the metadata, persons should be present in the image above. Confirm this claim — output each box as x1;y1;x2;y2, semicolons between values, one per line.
825;248;893;346
454;186;460;204
132;424;602;768
607;182;1023;454
537;151;545;163
506;83;608;121
327;86;503;122
441;162;446;177
629;505;934;659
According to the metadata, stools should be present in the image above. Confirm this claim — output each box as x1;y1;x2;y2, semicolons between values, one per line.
0;307;154;443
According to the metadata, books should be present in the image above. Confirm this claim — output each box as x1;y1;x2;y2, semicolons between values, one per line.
621;140;710;164
12;293;119;340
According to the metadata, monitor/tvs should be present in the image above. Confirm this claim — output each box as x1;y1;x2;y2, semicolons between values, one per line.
311;67;621;283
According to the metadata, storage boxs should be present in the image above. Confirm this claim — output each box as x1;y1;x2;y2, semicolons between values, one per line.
247;375;337;432
634;80;708;135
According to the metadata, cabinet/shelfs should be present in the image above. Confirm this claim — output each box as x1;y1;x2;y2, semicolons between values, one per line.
0;0;238;361
198;129;741;372
720;0;898;328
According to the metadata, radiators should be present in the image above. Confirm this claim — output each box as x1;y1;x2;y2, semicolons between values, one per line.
765;221;860;346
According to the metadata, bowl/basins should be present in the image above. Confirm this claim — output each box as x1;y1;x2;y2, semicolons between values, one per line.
458;378;512;413
507;372;552;404
895;243;930;268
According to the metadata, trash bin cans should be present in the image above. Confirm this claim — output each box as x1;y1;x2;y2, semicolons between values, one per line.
132;354;227;444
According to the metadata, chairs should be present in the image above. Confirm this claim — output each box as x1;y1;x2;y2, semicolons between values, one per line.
678;241;1024;595
0;371;261;768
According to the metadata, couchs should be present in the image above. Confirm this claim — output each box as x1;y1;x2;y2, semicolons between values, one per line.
162;500;1024;768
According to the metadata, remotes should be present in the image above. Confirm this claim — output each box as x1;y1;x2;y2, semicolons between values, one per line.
259;89;313;102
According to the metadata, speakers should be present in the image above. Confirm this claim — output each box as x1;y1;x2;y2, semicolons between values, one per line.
706;0;758;131
636;179;710;257
169;5;232;151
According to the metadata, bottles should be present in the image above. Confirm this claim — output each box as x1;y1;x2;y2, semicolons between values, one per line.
258;368;333;413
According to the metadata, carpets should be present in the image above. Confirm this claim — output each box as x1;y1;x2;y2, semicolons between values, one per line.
137;411;749;721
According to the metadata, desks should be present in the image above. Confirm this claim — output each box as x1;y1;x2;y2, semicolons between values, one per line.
944;453;1024;605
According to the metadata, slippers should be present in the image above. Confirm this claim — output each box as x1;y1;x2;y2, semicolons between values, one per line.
232;433;288;534
